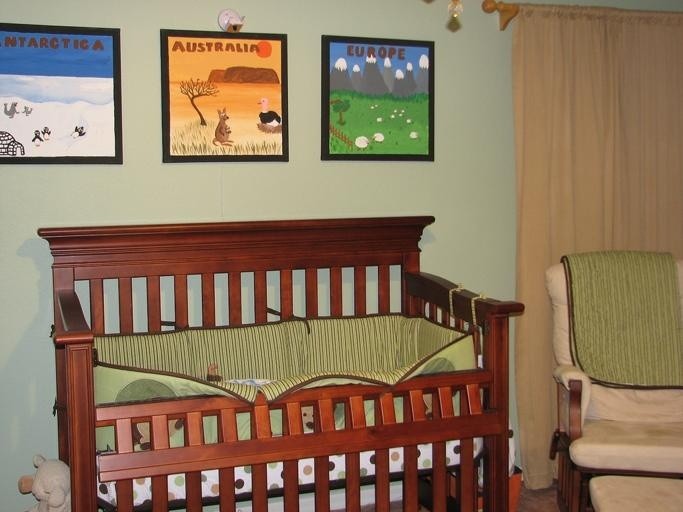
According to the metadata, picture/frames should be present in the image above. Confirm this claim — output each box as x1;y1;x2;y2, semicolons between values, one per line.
321;36;434;160
160;30;289;162
0;24;123;164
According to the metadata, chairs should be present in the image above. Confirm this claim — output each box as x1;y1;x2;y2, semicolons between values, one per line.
589;475;683;512
546;249;683;512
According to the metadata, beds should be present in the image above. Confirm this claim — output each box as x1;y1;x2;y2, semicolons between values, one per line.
39;217;525;512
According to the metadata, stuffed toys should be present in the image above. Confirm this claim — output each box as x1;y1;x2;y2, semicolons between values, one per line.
18;453;71;511
